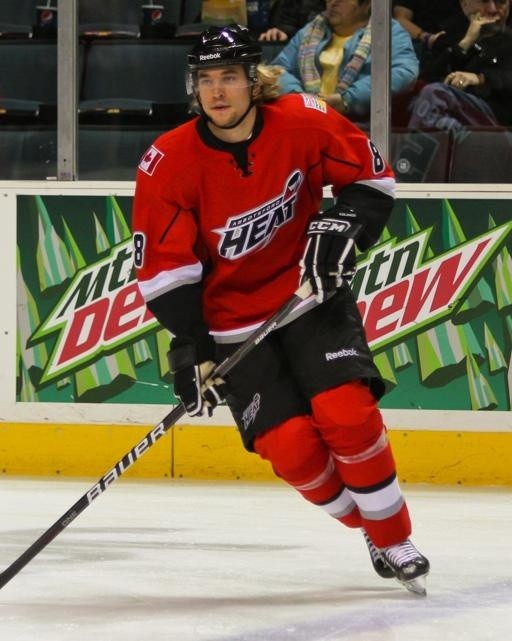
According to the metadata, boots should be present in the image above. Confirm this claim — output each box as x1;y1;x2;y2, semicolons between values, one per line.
360;524;430;582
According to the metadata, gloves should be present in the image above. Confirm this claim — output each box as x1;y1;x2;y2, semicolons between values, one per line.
298;205;367;304
166;336;229;418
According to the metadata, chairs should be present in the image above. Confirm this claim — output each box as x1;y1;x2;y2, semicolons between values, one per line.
361;117;509;185
0;0;190;180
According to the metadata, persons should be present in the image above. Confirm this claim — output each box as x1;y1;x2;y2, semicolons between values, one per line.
132;23;430;580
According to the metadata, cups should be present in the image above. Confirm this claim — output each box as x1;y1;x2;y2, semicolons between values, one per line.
141;0;164;24
35;0;58;31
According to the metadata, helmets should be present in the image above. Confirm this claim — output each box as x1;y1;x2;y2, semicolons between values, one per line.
187;23;263;70
